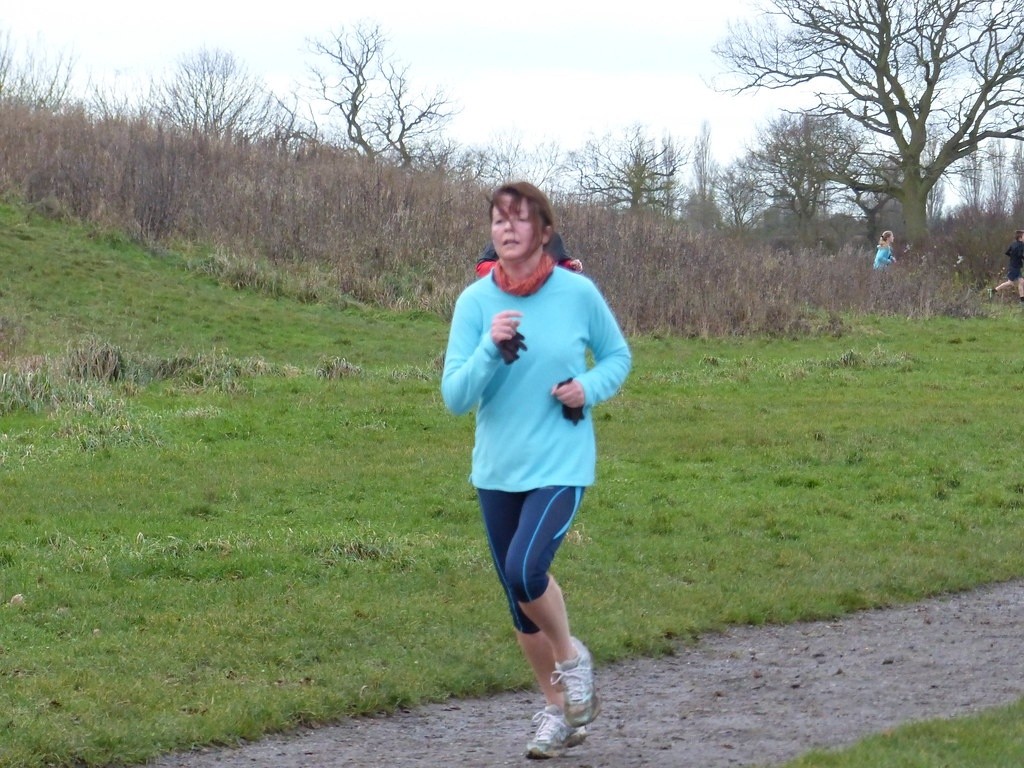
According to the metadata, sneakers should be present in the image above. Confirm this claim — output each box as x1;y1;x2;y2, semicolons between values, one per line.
524;705;587;759
549;634;599;727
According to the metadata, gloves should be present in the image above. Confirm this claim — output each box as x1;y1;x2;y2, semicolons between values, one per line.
559;377;585;425
497;330;527;364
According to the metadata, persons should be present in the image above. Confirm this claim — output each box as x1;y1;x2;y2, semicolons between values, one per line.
441;180;631;759
987;230;1024;303
873;231;896;271
